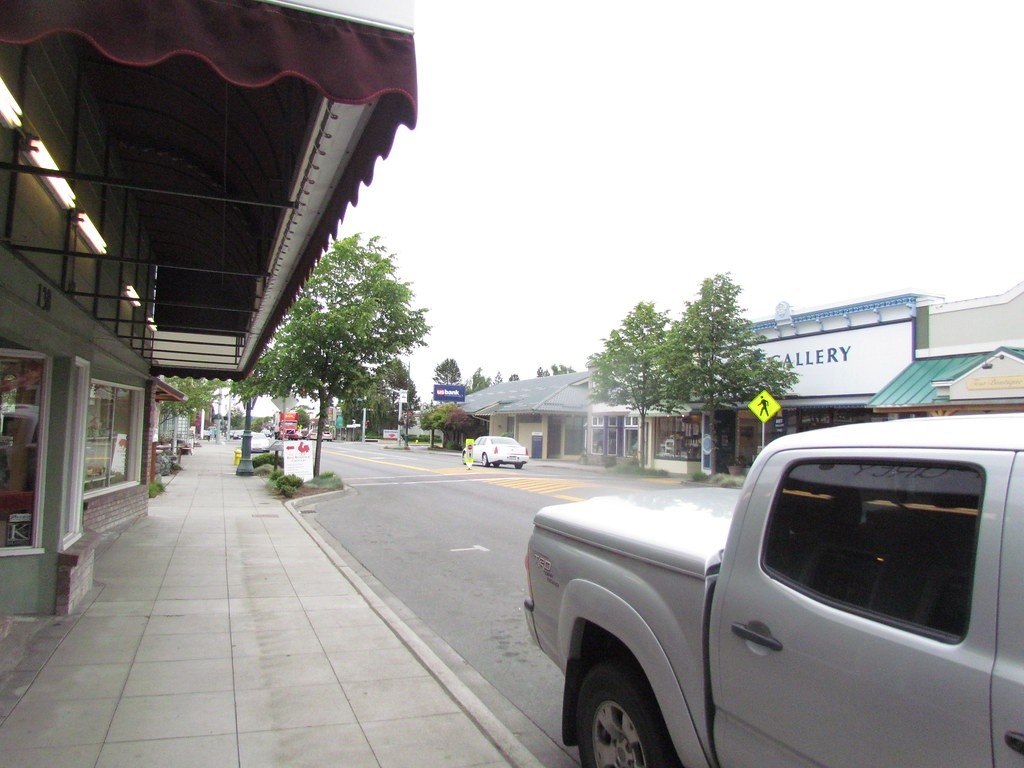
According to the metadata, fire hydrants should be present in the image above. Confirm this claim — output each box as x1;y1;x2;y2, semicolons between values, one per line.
234;449;243;465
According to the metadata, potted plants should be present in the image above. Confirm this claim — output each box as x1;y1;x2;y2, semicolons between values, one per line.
719;454;747;475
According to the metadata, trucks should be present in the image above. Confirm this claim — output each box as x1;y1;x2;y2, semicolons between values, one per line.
273;410;302;441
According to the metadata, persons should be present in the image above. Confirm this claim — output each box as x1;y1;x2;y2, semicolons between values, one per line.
214;428;217;438
207;428;210;441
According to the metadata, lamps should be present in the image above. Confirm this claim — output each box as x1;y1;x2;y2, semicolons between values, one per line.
69;209;108;255
145;317;158;332
982;354;1004;369
0;77;23;130
120;283;142;308
17;134;77;212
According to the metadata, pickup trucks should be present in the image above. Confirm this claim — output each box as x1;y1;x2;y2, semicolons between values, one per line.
517;410;1024;768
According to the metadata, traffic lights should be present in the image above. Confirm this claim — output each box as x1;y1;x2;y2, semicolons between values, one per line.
365;421;369;426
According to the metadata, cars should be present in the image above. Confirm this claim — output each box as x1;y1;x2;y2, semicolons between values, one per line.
461;434;529;469
0;401;41;488
300;429;334;441
224;427;274;455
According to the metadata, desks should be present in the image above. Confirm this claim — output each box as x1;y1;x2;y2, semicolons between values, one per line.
155;445;183;463
